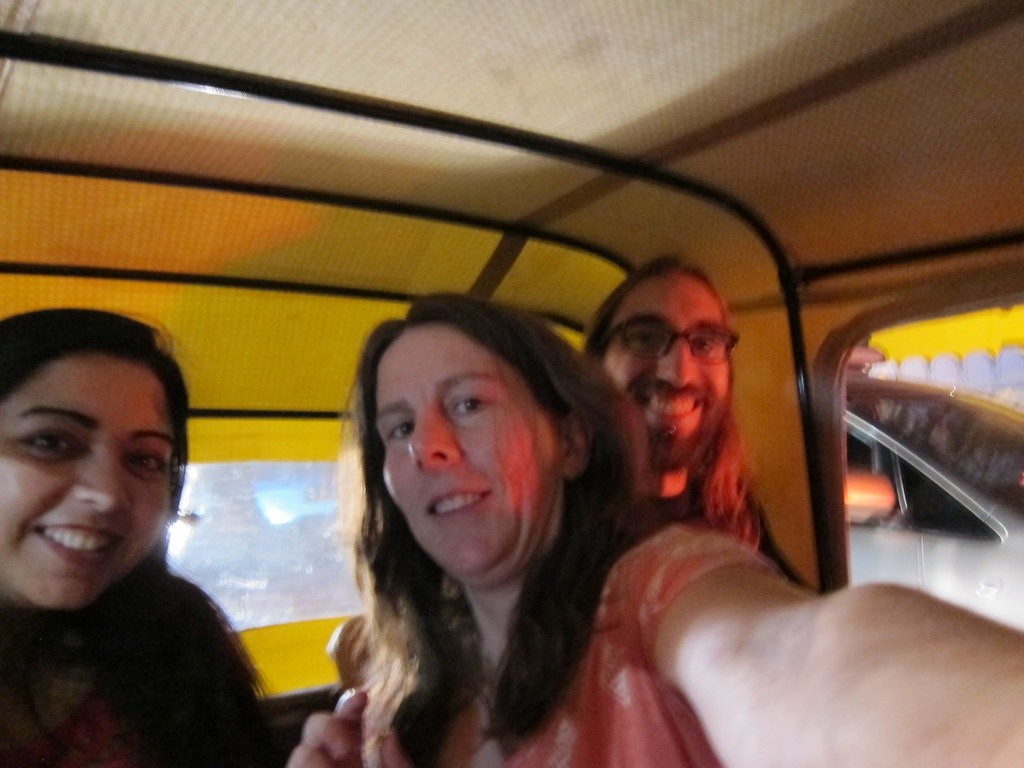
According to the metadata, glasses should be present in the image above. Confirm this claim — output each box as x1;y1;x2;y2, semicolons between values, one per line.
598;319;740;363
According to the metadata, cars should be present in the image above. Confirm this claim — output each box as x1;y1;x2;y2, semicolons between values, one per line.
846;376;1024;550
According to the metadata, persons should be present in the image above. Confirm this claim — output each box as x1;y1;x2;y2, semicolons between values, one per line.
286;255;1024;768
0;307;280;768
